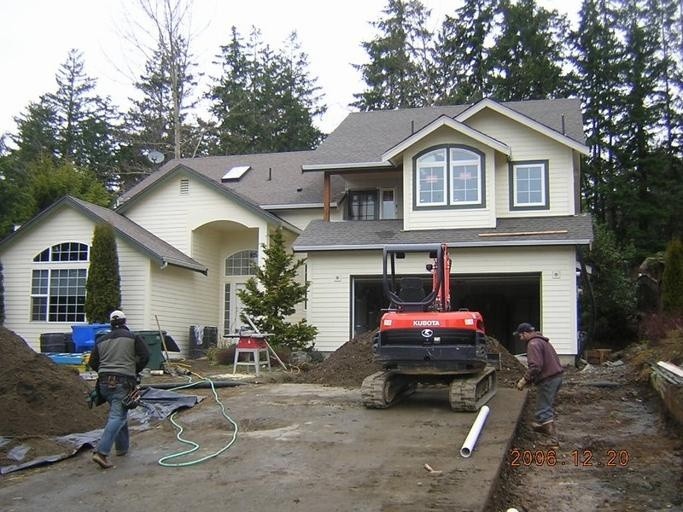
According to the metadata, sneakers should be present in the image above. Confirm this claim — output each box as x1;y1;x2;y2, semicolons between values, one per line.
116;450;128;456
92;453;113;468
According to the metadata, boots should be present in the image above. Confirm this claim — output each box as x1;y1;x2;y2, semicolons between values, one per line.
532;422;553;435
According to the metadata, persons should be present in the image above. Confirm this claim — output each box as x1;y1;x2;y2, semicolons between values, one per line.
88;309;151;469
512;322;563;434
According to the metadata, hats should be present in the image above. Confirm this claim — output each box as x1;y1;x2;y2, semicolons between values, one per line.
110;310;126;321
513;323;534;336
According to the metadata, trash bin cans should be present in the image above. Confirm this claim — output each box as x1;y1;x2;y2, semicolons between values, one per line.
203;326;218;348
133;331;167;370
41;333;75;353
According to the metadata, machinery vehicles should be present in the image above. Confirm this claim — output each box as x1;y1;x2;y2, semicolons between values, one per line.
360;244;501;412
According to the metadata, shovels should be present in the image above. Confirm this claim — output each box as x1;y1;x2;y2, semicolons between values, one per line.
155;315;178;377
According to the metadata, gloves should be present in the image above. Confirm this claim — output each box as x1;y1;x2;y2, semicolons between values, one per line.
515;377;528;391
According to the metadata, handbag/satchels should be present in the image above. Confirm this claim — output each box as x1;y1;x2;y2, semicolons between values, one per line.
122;388;140;409
84;378;106;409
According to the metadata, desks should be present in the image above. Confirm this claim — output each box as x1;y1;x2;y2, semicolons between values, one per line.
224;334;273;377
